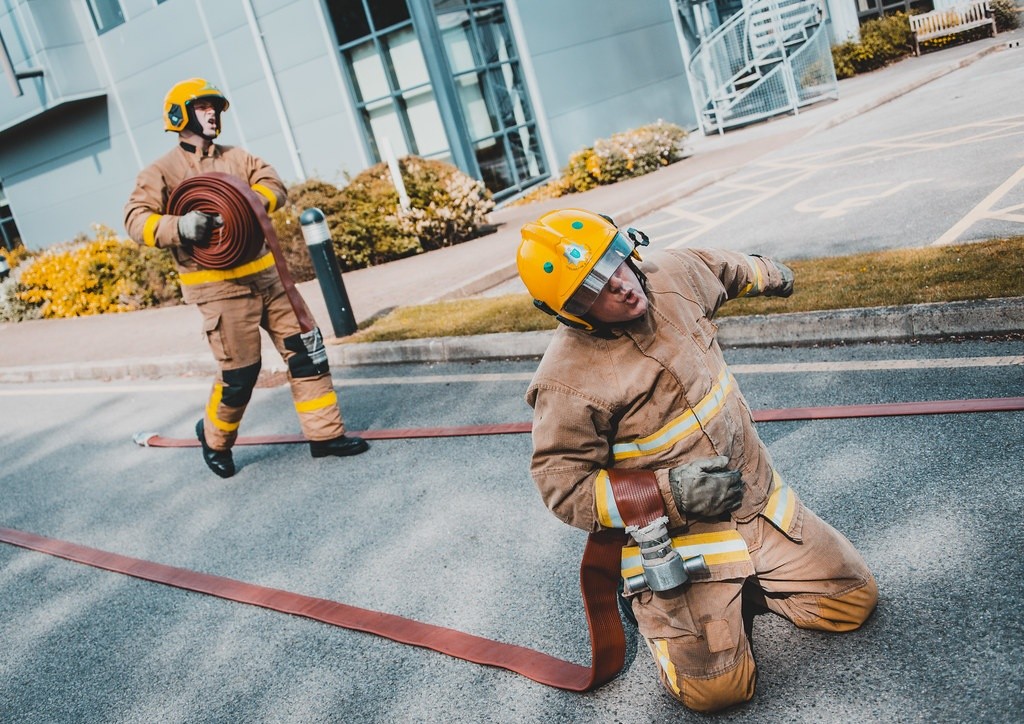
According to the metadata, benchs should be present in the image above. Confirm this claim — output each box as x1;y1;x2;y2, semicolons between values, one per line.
908;0;997;56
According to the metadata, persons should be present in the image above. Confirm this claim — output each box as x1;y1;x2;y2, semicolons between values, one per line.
124;77;371;478
514;209;880;709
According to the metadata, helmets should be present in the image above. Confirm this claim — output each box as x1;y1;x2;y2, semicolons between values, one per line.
163;78;229;136
517;209;643;330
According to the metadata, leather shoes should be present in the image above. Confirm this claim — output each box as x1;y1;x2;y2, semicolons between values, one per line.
310;434;368;458
195;419;236;478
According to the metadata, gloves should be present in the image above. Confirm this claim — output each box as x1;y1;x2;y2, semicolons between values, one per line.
774;261;795;297
177;210;213;242
670;455;745;517
